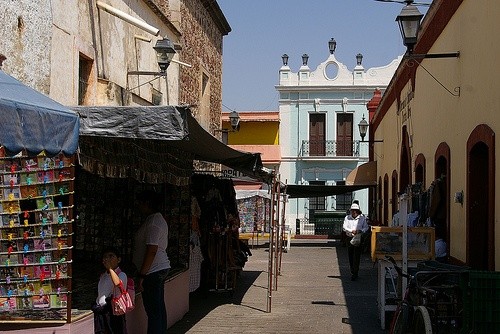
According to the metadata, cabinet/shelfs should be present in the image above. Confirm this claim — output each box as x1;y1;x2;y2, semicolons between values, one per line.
377;259;418;329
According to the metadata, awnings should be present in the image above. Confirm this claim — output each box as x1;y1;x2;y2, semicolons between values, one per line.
248;166;287;311
0;69;80;155
65;104;263;180
345;160;377;186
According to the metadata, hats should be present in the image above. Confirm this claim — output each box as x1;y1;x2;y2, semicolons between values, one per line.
351;203;360;209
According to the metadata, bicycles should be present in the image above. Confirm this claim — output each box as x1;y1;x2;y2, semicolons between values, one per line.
383;254;453;334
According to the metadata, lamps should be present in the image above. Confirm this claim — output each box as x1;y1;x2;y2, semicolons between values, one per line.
356;53;363;65
302;54;309;66
126;35;176;92
214;111;240;136
328;38;336;54
282;53;289;66
358;113;384;159
395;0;461;96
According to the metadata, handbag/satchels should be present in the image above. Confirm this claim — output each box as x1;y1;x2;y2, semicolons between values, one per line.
111;279;135;316
349;230;362;247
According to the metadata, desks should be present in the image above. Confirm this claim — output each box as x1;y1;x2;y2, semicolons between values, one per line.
153;269;190;329
0;309;96;334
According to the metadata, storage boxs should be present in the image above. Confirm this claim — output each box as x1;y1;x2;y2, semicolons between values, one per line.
371;225;435;261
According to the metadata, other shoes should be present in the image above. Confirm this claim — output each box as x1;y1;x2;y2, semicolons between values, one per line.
350;273;358;282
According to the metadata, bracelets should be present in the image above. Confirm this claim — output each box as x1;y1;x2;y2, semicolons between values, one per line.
138;273;146;279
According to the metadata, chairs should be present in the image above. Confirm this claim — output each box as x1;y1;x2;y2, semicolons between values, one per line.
207;237;242;289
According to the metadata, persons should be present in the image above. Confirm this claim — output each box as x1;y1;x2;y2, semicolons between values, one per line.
131;191;172;334
342;203;369;281
94;247;127;334
